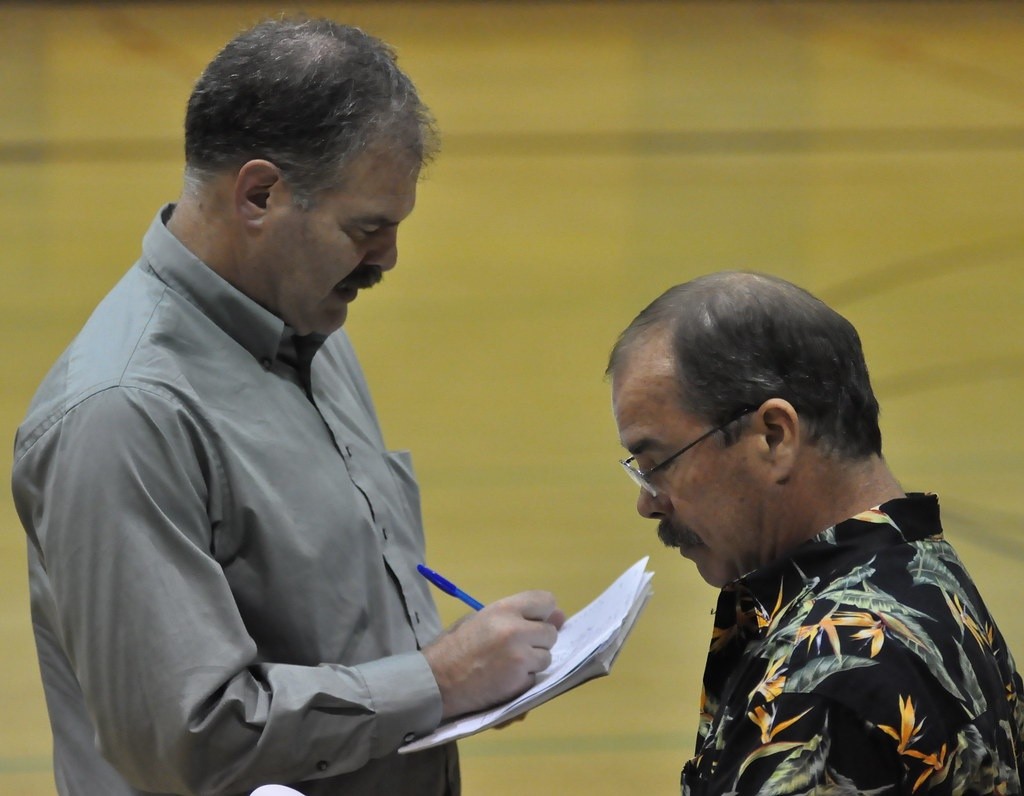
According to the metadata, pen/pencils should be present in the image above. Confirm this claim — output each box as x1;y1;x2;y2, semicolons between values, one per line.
417;563;484;610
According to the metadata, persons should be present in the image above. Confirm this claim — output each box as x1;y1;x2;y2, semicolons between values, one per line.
1;16;562;796
603;271;1024;795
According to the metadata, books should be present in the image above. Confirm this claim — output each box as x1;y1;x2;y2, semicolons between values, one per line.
398;555;655;755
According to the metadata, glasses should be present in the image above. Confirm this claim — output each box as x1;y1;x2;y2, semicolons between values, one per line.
620;404;799;498
